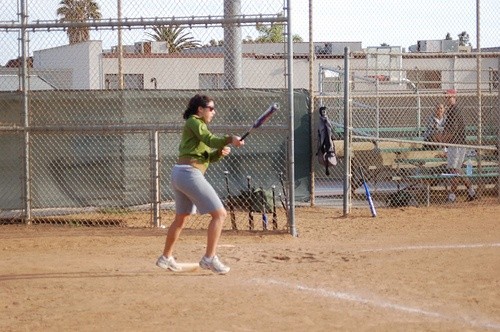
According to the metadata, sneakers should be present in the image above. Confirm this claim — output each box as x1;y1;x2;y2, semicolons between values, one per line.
199;254;230;275
155;255;184;272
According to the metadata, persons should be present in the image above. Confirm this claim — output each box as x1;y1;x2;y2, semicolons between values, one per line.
156;93;244;274
435;89;478;204
422;101;447;151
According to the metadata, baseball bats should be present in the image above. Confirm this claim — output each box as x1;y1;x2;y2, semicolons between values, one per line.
279;192;286;210
247;175;254;229
272;185;278;230
260;185;268;230
279;172;288;209
240;103;280;141
359;166;377;217
224;171;237;230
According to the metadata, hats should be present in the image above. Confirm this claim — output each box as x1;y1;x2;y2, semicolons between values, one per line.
444;89;458;97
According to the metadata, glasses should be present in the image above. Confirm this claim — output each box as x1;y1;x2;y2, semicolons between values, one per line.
205;106;214;112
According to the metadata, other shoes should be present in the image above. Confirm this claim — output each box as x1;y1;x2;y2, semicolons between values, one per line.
442;197;458;205
464;194;478;202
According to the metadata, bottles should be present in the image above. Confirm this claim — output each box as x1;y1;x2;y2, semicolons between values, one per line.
467;157;472;175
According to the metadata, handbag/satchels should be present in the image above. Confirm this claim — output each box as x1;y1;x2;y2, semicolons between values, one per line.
241;188;274;213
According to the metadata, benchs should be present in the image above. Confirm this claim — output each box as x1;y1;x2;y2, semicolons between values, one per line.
333;126;500;206
